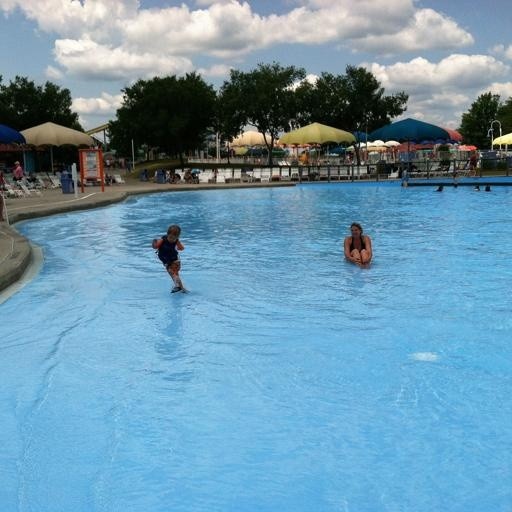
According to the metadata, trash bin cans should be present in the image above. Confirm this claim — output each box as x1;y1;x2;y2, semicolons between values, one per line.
61;174;74;194
155;169;166;183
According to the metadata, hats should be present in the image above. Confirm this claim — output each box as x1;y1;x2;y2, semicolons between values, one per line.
14;160;20;165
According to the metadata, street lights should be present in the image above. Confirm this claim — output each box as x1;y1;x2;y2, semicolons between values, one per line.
487;129;494;150
491;120;503;150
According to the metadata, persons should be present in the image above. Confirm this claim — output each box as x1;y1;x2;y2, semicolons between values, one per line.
12;160;22;182
0;194;5;222
344;222;372;269
153;225;188;293
106;158;109;168
1;171;8;191
140;168;199;184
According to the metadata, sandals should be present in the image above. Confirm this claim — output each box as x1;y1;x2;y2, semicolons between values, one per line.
170;286;186;294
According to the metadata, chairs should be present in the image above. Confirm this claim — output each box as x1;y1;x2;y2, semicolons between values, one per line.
172;160;474;184
2;166;124;199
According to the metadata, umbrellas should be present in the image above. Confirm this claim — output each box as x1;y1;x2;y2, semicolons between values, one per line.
18;121;94;173
273;129;476;159
226;130;278;156
367;118;450;161
493;132;512;146
277;122;356;163
0;123;25;145
